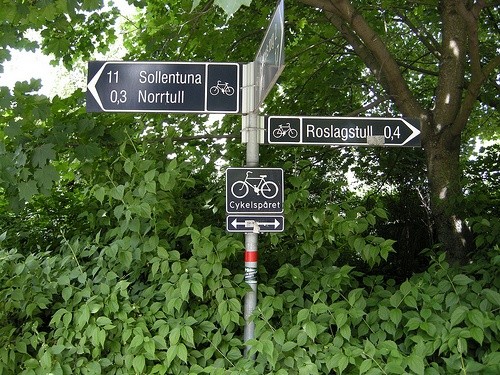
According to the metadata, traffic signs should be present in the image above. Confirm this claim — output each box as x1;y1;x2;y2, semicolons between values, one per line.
267;116;421;146
86;59;241;117
253;0;285;111
224;166;285;234
